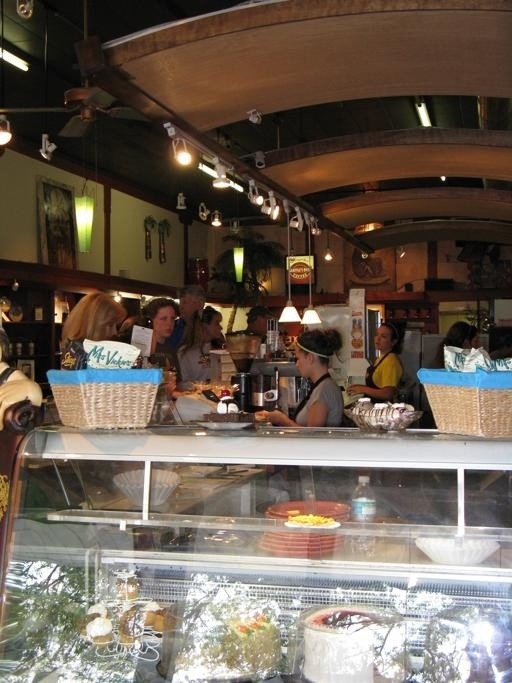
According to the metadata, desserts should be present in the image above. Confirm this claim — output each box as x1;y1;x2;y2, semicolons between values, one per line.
77;573;177;648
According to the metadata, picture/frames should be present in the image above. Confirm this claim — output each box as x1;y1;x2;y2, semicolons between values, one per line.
36;175;78;271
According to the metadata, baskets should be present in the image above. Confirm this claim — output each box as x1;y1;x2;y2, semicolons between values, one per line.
414;366;512;440
46;366;164;431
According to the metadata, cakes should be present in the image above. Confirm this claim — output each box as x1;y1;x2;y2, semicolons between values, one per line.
302;604;406;683
221;612;282;672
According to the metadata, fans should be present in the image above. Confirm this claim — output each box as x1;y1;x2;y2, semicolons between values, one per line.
0;0;151;137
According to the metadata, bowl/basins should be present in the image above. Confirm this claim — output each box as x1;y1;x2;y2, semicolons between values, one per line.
413;537;502;567
342;409;424;434
111;469;181;506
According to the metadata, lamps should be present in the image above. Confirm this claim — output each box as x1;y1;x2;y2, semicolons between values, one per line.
75;196;94;253
0;115;13;145
278;208;302;323
300;224;322;325
163;123;321;236
40;134;56;160
233;247;245;282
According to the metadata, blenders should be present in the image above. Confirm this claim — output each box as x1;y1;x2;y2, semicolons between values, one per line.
223;331;276;414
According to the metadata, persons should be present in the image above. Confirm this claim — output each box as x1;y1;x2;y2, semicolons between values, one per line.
114;313;159;358
253;328;345;501
434;319;480;367
246;306;280;338
176;306;224;386
142;297;195;400
343;320;406;495
56;289;129;357
165;283;206;349
1;323;30;381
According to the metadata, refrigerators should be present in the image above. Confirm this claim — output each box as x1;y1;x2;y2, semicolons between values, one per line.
301;304;378;389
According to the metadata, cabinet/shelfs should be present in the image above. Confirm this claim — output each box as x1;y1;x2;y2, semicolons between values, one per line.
0;425;512;625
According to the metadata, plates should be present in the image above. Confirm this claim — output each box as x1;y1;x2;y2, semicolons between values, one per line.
161;529;255;554
259;500;351;560
188;419;255;431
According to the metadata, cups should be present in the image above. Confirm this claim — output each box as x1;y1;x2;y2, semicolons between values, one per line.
189;257;210;293
255;343;272;359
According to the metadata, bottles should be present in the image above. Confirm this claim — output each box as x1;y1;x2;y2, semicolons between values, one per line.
7;342;40;384
385;302;430;319
351;474;378;562
